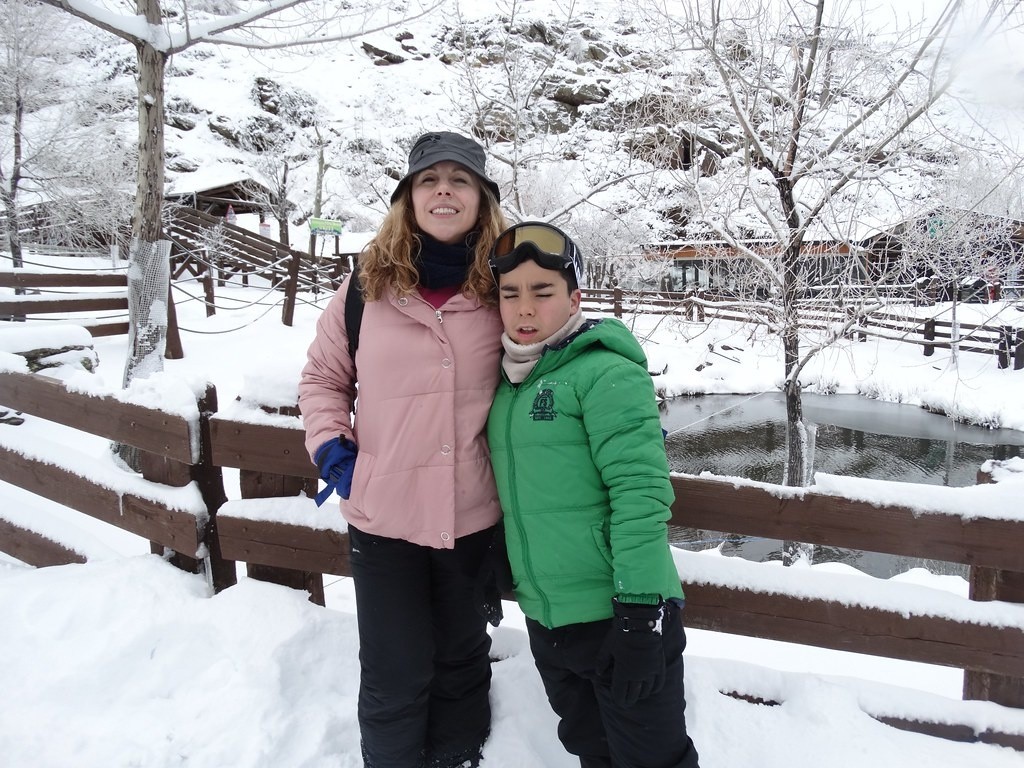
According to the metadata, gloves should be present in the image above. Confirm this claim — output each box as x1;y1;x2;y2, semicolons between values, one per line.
595;595;666;708
314;434;358;508
483;531;511;595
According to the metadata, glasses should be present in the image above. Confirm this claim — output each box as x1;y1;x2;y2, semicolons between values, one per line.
488;221;581;289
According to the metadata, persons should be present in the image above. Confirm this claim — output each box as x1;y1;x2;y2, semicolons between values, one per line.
488;220;700;768
299;129;513;768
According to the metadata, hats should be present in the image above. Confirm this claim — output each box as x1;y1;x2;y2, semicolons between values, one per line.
391;131;500;205
